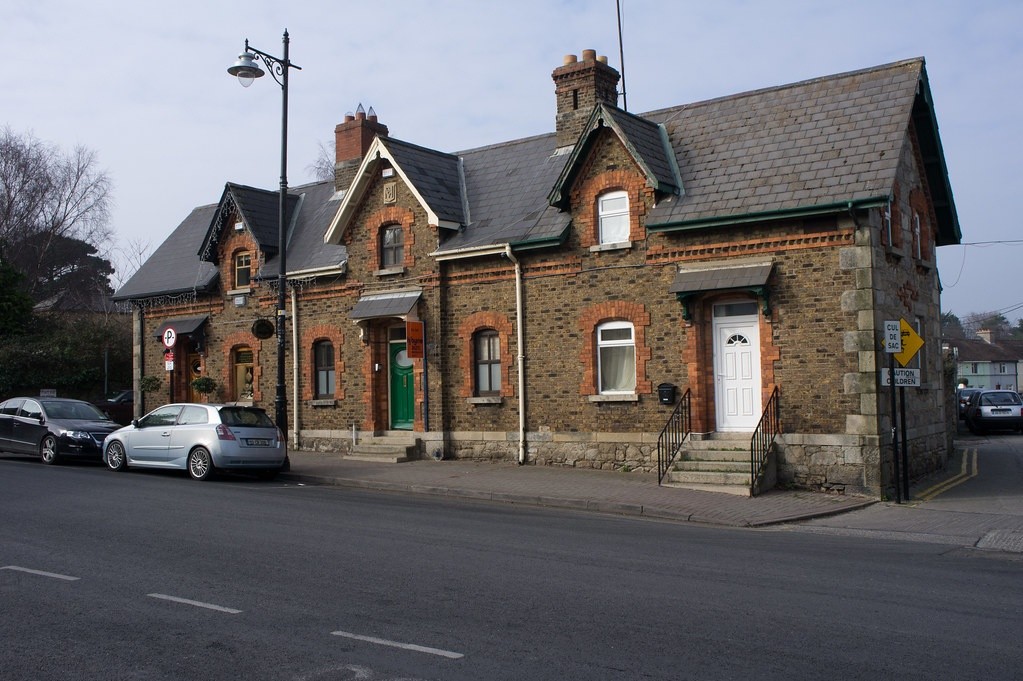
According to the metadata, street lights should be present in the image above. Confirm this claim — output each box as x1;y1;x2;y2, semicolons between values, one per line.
227;28;302;472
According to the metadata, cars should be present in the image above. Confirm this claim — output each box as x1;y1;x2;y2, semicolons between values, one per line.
957;388;981;419
102;403;286;481
964;389;1023;435
0;396;123;465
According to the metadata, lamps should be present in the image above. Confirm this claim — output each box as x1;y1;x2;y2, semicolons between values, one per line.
761;291;771;318
682;300;692;321
